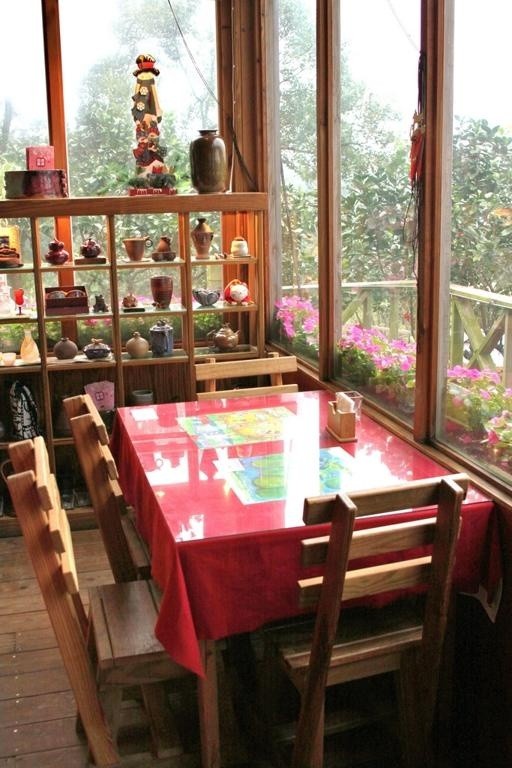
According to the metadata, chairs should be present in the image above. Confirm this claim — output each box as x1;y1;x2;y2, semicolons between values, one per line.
62;394;151;583
260;473;472;768
192;352;299;401
7;437;240;767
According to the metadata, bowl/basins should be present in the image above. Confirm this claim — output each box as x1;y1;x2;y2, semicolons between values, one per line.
192;287;221;306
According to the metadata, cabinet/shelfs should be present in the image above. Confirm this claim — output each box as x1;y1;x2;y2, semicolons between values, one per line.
0;191;269;539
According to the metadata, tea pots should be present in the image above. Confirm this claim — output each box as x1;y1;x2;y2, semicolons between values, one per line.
80;238;102;258
206;322;241;350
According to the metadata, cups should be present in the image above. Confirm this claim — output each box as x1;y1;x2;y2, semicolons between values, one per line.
66;290;85;297
151;252;177;262
48;291;68;299
122;235;153;261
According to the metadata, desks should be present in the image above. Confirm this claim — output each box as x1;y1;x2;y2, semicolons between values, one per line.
110;388;494;768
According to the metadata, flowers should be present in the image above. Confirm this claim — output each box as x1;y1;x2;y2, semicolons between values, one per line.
446;364;512;475
270;295;319;357
335;323;416;411
